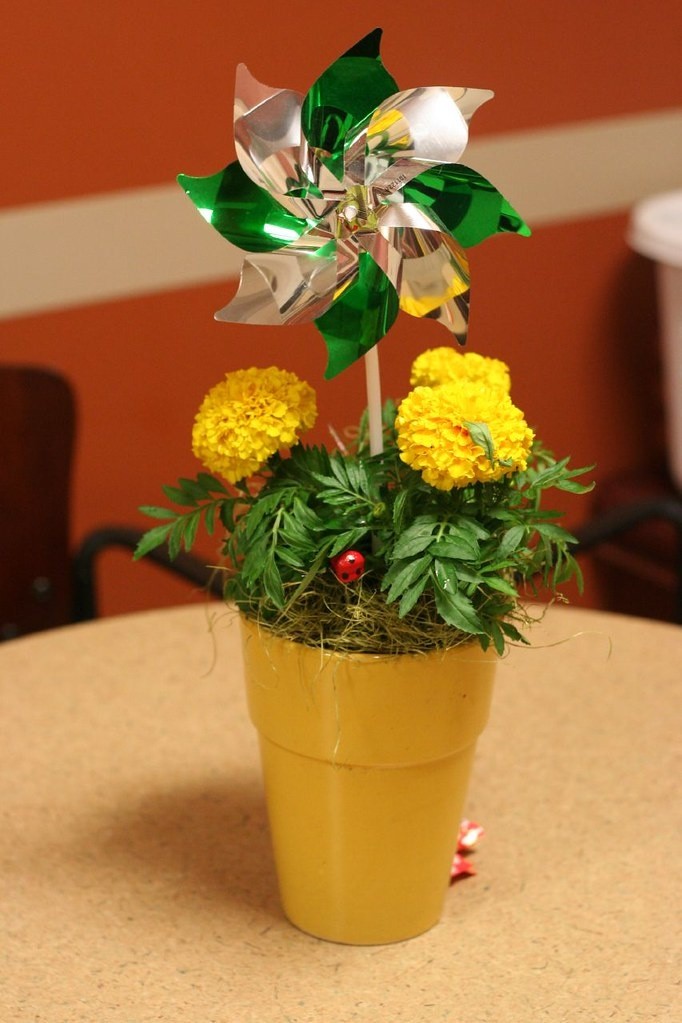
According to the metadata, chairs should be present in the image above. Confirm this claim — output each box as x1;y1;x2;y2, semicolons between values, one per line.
0;361;226;635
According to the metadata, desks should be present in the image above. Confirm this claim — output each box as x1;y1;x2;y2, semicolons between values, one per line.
0;606;682;1023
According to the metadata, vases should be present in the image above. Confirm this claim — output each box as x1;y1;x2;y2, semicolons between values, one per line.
238;612;502;947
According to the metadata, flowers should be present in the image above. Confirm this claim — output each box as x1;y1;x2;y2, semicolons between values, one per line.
133;346;600;658
